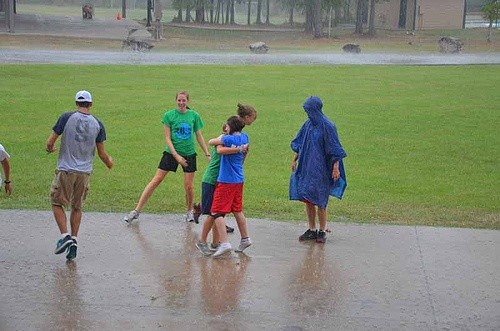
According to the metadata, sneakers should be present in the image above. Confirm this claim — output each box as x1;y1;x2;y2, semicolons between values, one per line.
124;209;139;223
316;229;326;243
184;214;194;223
66;239;77;259
193;202;201;224
299;229;317;240
208;241;219;251
212;247;231;259
234;240;252;252
226;225;234;232
194;240;213;256
54;235;73;254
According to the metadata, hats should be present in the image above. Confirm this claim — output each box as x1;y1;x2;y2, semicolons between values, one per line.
75;89;92;102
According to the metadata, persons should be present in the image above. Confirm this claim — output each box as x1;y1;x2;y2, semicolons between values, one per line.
291;95;340;243
225;103;256;233
194;124;231;256
0;143;12;195
124;90;211;224
208;116;252;258
46;90;113;259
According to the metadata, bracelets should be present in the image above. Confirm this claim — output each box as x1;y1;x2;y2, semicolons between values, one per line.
205;154;210;157
5;180;11;184
239;146;242;154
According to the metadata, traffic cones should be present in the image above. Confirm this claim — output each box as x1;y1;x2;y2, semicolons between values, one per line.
117;12;121;20
